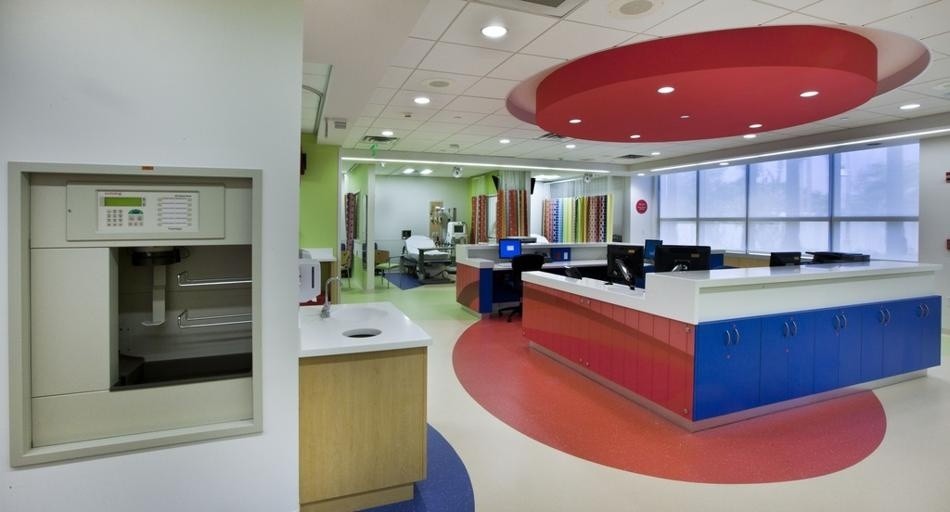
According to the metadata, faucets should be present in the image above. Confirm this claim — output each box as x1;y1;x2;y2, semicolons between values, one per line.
320;276;343;318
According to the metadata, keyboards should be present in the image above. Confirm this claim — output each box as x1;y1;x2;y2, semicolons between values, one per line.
498;262;512;267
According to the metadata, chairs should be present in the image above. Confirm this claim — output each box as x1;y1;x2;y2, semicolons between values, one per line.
498;253;544;323
341;249;350;289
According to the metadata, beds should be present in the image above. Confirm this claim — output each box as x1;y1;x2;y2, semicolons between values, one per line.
401;235;456;285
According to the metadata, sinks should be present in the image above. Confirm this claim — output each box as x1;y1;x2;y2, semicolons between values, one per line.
342;327;382;338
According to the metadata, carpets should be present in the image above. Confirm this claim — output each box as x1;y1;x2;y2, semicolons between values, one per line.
379;272;424;291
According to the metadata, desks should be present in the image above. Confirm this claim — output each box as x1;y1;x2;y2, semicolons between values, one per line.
454;241;654;318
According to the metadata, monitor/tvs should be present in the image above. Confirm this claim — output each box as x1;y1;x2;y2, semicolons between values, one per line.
769;252;801;267
644;239;662;265
605;245;645;289
400;228;414;240
499;239;521;262
454;226;463;232
655;245;711;271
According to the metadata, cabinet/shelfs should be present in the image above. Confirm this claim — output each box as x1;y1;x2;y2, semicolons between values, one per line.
694;294;941;424
